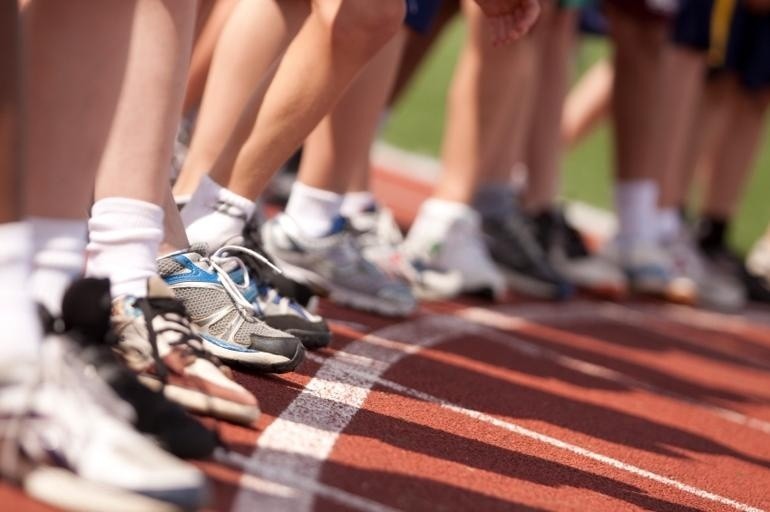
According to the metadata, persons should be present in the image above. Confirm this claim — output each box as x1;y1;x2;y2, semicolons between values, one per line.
0;1;769;511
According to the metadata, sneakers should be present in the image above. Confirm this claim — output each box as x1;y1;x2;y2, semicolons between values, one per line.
160;210;335;372
257;209;417;318
0;337;204;512
110;285;262;427
400;199;770;311
34;278;215;459
